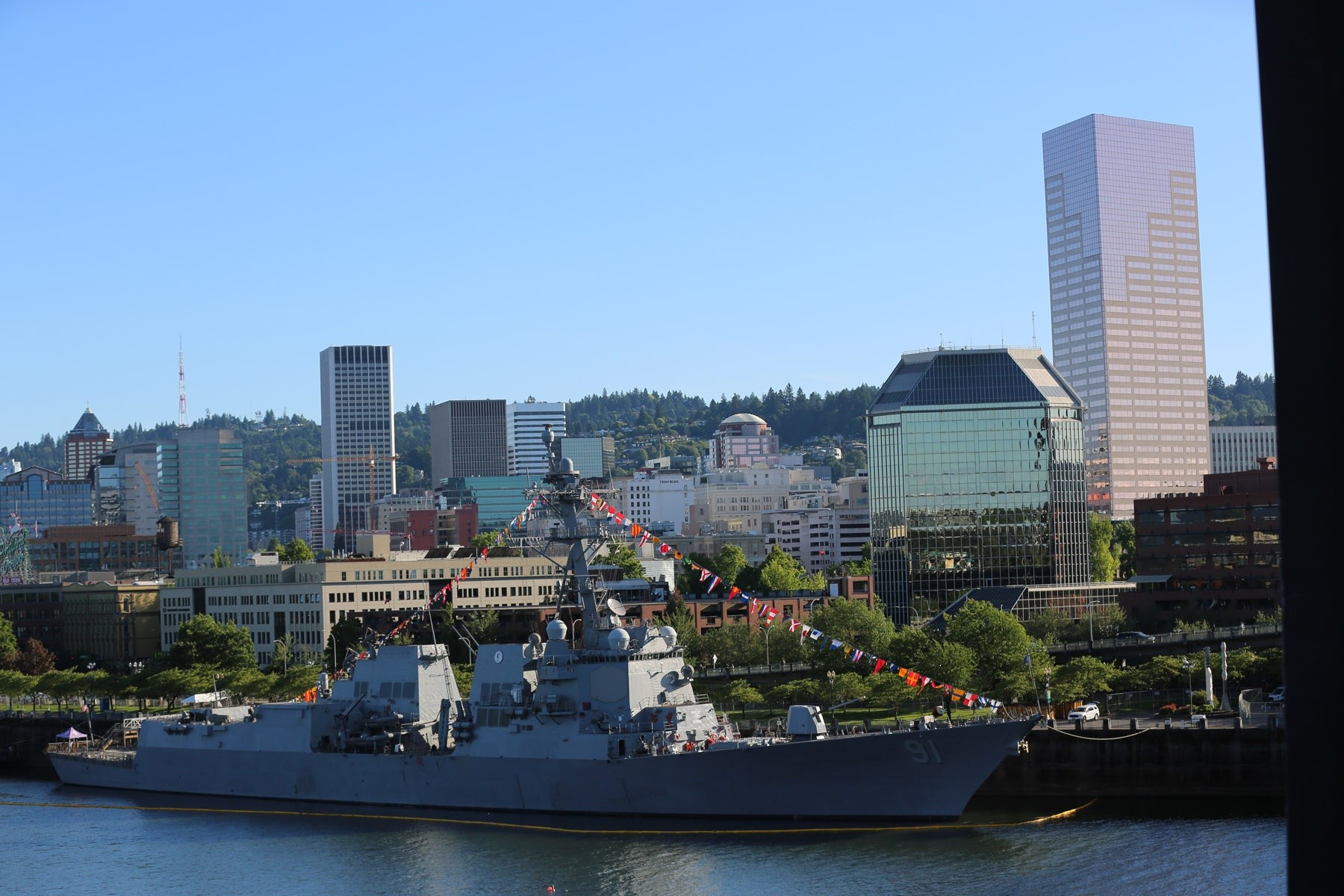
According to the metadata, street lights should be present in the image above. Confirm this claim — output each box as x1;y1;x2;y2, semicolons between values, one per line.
273;640;287;676
321;631;336;674
1182;660;1193;717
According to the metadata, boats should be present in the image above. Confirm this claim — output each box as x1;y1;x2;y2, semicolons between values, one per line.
48;419;1045;825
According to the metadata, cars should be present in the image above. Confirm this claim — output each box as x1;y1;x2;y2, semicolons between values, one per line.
1068;703;1100;722
1268;686;1285;702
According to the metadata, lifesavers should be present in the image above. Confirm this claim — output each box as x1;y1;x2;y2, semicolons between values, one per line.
712;733;718;741
674;735;679;741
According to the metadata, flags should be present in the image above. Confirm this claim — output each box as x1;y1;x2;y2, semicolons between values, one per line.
830;637;1002;713
728;587;823;645
591;493;721;593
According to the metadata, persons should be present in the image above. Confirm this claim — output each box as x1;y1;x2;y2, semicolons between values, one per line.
897;719;904;730
781;657;787;670
698;664;708;679
1212;624;1216;638
725;662;735;676
1240;623;1245;635
1050;708;1054;721
1069;707;1072;713
852;725;859;735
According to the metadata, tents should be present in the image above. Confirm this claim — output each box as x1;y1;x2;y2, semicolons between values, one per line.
181;687;242;723
56;726;88;753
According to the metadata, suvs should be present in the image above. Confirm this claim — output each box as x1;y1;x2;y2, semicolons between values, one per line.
1114;631;1155;645
